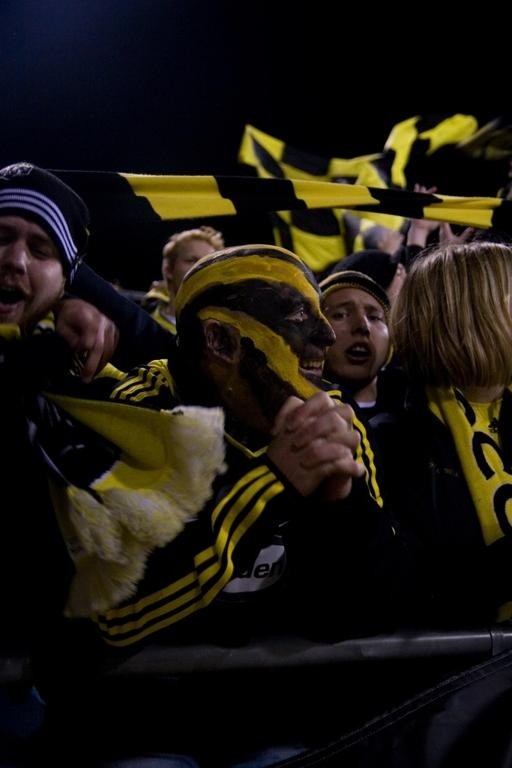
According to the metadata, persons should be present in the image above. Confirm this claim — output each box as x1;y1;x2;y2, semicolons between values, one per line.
2;162;512;768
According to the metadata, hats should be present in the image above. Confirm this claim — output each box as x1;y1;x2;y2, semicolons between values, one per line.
1;162;85;284
320;268;390;318
